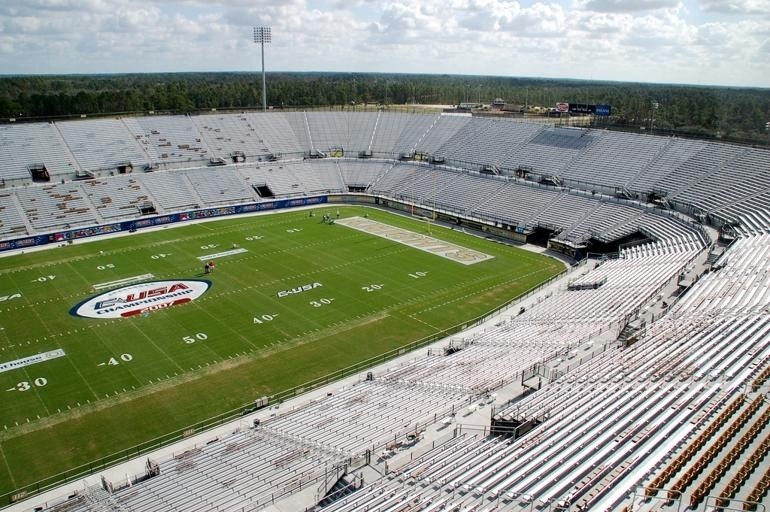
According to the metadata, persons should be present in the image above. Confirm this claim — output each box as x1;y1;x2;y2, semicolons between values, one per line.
204;260;216;273
308;209;340;225
